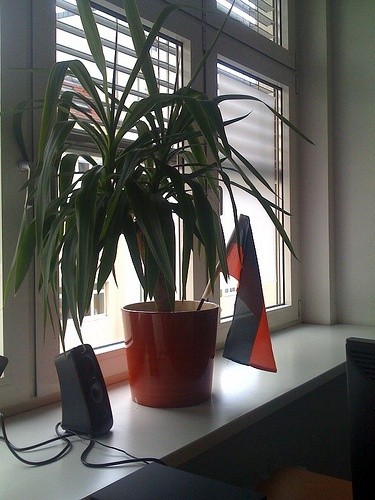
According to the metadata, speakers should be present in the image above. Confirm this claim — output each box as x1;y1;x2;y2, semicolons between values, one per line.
55;344;113;436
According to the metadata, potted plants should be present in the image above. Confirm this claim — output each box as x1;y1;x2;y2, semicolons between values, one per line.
9;0;317;409
